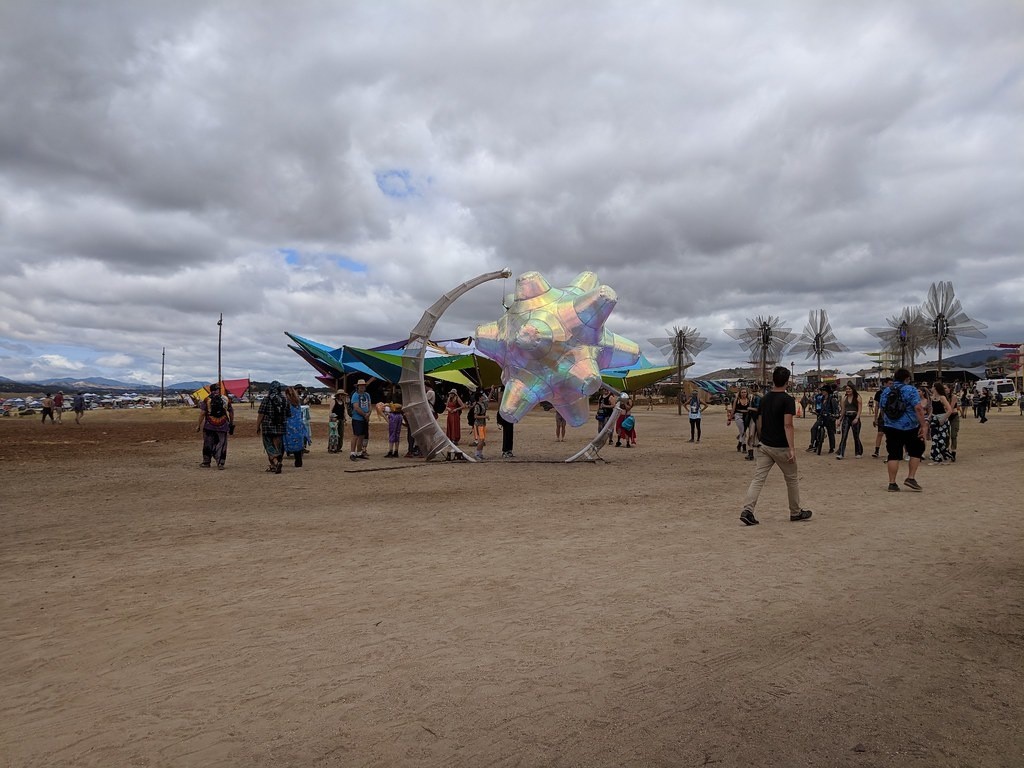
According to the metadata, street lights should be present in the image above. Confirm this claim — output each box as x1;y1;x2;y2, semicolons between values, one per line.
790;361;795;395
216;313;223;395
162;347;165;409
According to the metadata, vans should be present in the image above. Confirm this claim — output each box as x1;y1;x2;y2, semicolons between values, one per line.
966;378;1017;406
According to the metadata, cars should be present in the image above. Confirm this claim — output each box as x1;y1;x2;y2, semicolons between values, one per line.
708;391;737;405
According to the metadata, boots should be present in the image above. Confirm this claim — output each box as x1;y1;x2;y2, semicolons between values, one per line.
736;442;742;452
742;444;749;453
745;449;754;460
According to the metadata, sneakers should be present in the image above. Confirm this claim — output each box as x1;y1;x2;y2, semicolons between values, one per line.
888;484;898;492
903;478;922;489
790;509;812;521
740;510;759;525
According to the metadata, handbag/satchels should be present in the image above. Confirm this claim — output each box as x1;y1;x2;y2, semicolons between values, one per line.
621;416;635;431
390;404;402;413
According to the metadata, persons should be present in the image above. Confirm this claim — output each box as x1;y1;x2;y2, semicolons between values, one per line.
555;410;567;442
257;381;312;474
74;392;84;424
640;388;660;410
727;384;763;460
464;383;490;459
597;385;637;448
196;385;235;470
250;393;255;408
741;367;813;524
42;393;55;424
425;380;438;419
868;369;1003;492
328;379;372;461
385;404;424;458
502;422;514;458
54;391;64;423
809;380;864;459
683;390;708;442
446;389;465;445
1017;392;1024;415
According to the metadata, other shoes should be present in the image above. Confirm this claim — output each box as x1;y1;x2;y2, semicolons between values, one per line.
615;442;622;446
626;442;630;447
608;440;613;445
905;452;910;460
921;454;927;460
856;454;864;458
200;441;517;482
836;455;843;459
872;452;880;457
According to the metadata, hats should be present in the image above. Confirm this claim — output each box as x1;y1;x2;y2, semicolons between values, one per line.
353;379;367;386
448;388;458;396
334;389;349;397
820;385;831;393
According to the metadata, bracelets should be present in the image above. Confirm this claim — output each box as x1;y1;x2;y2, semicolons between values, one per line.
728;419;731;421
856;417;859;419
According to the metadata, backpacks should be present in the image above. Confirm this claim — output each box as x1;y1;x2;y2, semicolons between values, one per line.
466;403;483;425
347;391;356;417
883;384;906;420
428;390;446;414
